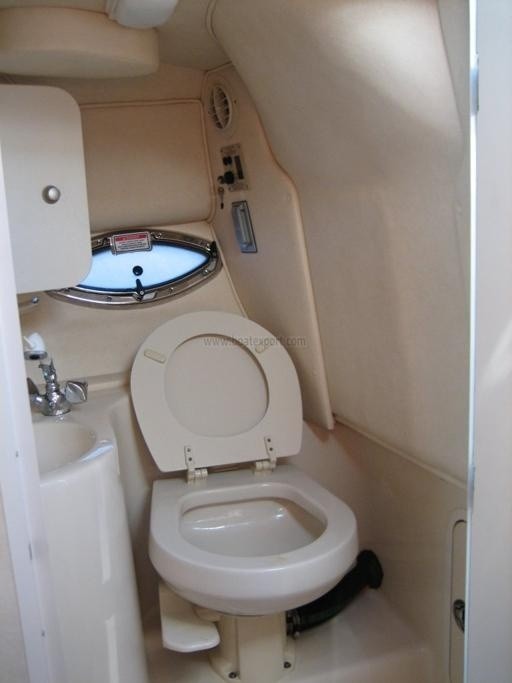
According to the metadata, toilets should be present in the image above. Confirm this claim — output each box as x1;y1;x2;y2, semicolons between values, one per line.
128;311;358;683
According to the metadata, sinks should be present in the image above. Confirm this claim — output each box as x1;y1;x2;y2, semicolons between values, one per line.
28;412;100;482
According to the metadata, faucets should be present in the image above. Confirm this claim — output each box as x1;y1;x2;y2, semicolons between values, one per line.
23;329;86;416
